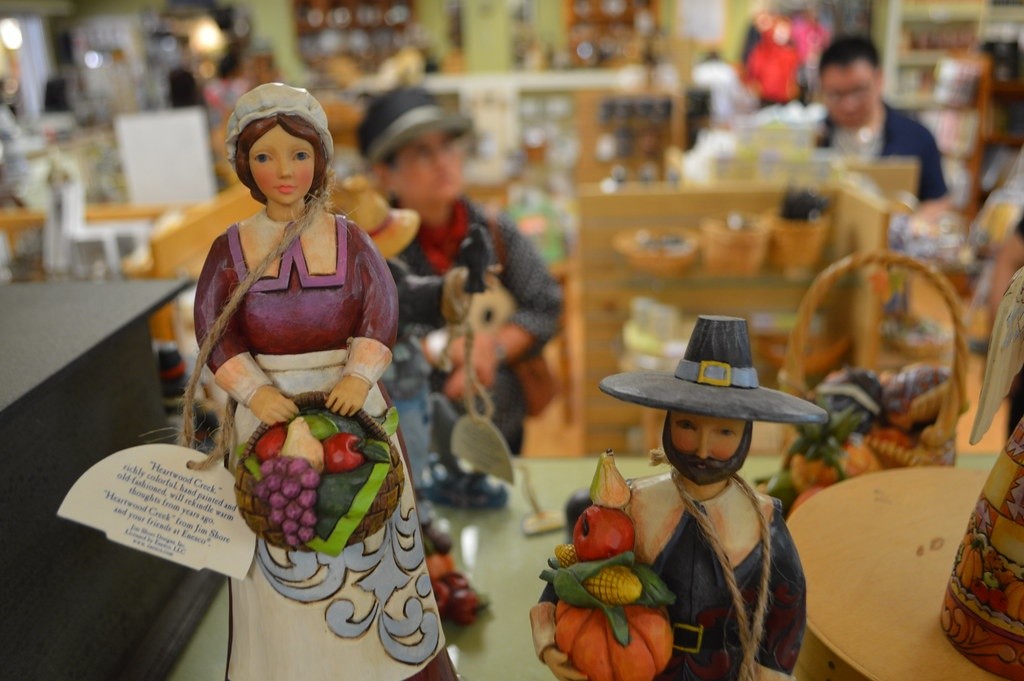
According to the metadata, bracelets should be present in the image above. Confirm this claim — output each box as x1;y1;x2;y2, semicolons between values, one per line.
428;331;450;372
492;337;505;368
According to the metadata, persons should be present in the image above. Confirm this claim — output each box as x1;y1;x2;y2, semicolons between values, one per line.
530;314;828;681
331;176;493;629
741;4;831;108
615;47;682;96
691;48;760;129
367;46;437;77
167;50;250;129
780;35;949;220
354;87;561;459
193;83;459;681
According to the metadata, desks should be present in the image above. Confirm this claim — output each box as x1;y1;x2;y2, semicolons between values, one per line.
170;454;1001;680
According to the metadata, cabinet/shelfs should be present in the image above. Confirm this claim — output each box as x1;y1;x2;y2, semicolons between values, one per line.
576;160;918;453
968;80;1024;221
875;0;985;109
292;0;416;72
563;0;660;70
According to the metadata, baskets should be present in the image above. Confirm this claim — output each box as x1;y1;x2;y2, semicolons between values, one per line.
778;251;966;468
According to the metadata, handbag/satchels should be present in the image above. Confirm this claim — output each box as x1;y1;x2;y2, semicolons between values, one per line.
515;354;552;413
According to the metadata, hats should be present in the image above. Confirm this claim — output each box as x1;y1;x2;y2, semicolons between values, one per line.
356;88;473;164
